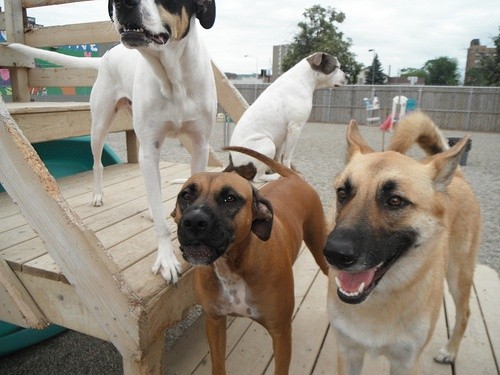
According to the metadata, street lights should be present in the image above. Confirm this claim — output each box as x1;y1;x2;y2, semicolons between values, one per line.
369;49;376;84
244;54;259;84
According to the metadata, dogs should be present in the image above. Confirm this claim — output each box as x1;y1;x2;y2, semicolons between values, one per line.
164;52;351;186
322;110;483;375
0;0;217;289
170;146;326;375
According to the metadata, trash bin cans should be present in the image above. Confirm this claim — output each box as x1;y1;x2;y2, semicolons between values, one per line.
448;136;473;165
